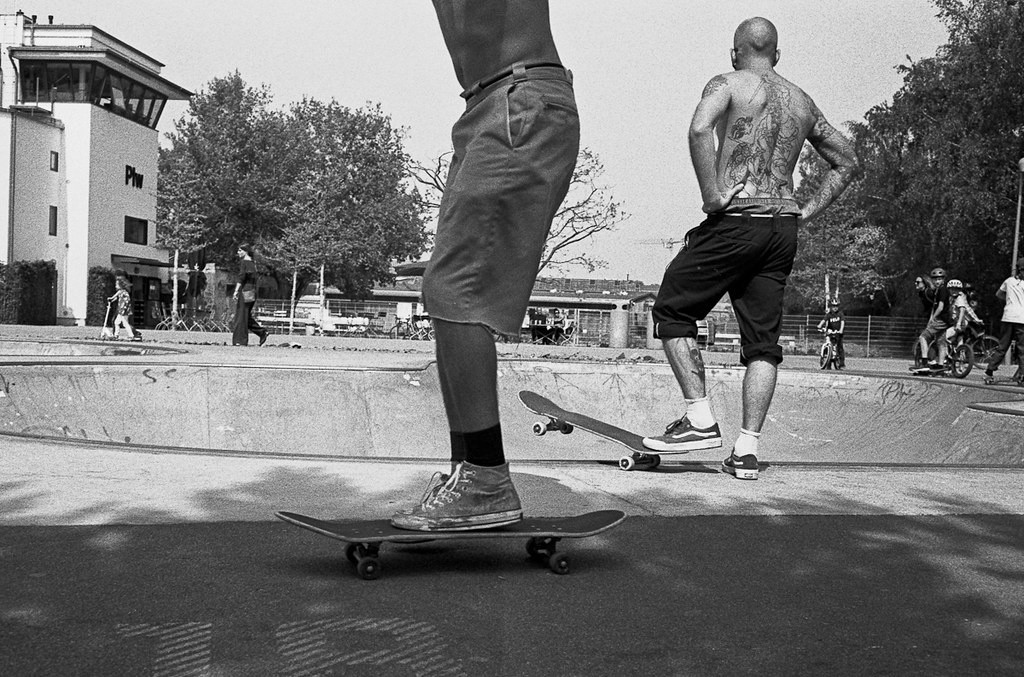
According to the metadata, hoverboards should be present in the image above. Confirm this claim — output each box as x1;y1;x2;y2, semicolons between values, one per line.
97;298;144;343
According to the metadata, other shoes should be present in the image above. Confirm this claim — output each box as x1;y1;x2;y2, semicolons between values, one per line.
929;367;946;373
839;363;845;367
984;370;993;377
391;459;525;530
259;331;269;347
909;366;929;373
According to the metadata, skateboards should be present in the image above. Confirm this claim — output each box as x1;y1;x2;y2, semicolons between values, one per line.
272;509;630;581
517;390;691;471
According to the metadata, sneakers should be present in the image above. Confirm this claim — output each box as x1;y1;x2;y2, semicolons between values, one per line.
721;451;760;481
643;412;724;452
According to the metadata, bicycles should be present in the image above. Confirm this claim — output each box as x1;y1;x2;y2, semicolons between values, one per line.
913;317;1001;378
387;312;435;340
817;328;841;370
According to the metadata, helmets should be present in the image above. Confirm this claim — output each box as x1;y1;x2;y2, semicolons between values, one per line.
829;297;841;305
930;268;947;277
916;277;923;282
947;279;963;288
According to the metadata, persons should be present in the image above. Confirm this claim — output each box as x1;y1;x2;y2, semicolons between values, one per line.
918;267;950;369
982;258;1024;385
816;296;846;368
642;16;859;479
546;308;565;343
107;278;134;338
915;275;935;316
232;242;269;347
389;0;580;530
929;279;968;365
566;321;577;335
183;260;208;306
112;268;141;336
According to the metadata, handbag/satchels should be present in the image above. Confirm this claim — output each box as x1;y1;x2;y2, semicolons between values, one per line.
242;289;257;303
